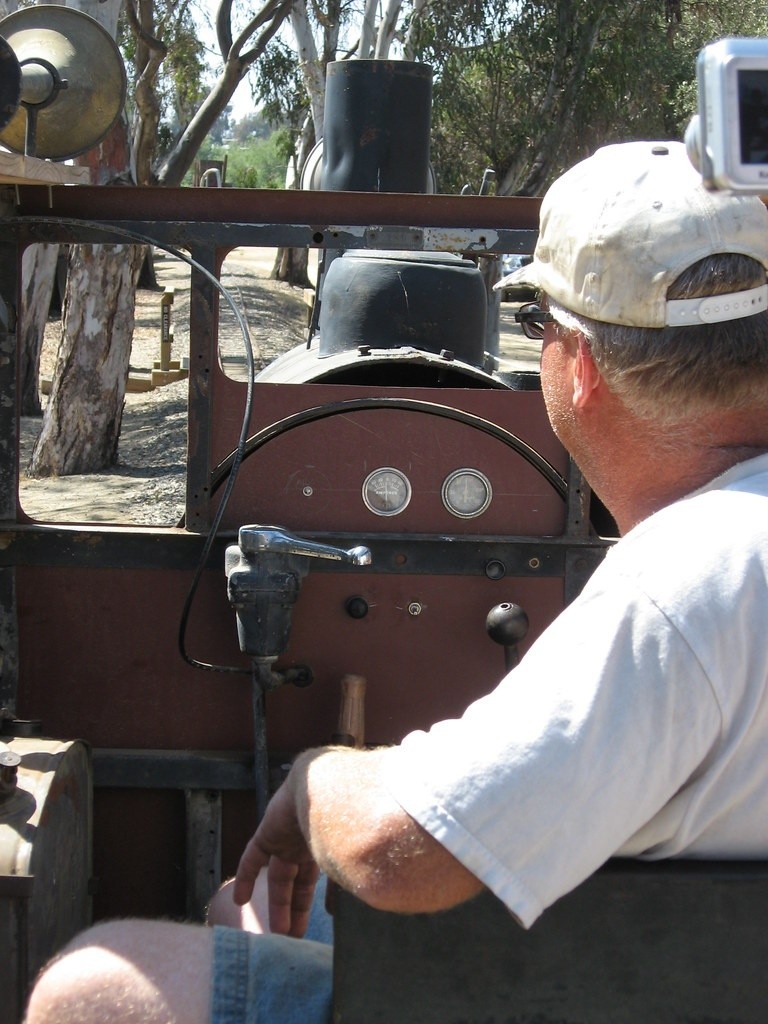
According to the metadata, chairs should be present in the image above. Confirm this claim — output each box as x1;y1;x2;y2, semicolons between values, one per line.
333;860;767;1024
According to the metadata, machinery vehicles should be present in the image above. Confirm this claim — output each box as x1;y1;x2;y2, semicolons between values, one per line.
297;136;440;328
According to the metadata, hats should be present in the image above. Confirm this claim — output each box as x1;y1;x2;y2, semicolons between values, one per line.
493;141;768;328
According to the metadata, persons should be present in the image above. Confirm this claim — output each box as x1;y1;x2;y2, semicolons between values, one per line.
17;141;768;1024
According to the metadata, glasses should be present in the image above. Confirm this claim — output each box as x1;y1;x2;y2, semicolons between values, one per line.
515;301;559;340
